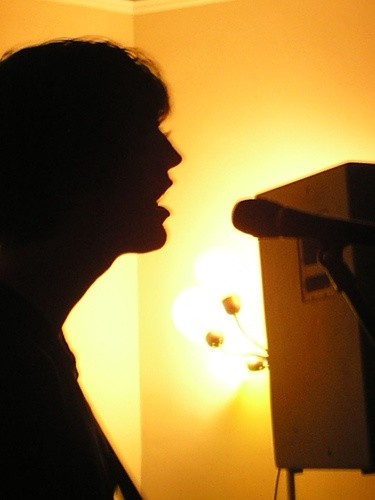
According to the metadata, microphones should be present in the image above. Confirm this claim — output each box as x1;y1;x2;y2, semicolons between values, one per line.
232;200;375;247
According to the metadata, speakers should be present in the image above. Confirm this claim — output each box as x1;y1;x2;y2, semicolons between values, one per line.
256;163;375;474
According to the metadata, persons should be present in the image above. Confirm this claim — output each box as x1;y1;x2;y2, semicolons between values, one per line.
0;37;183;499
